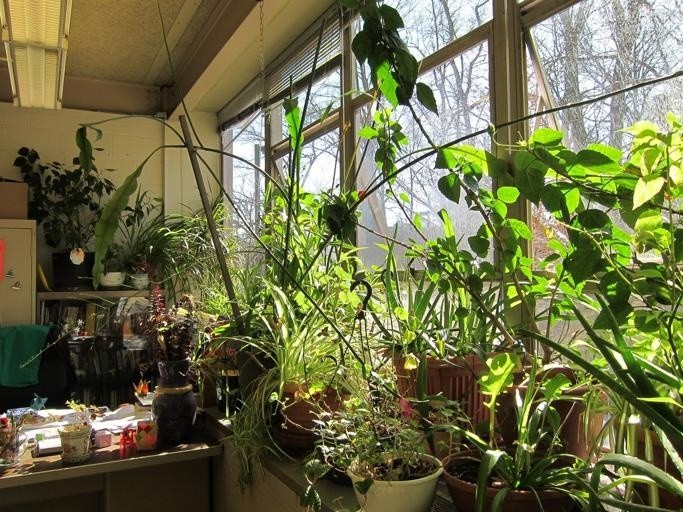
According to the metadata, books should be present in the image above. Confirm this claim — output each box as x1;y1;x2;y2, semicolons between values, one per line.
38;438;62;455
41;300;123;335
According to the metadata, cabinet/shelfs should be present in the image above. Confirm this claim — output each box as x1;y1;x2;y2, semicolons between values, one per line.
0;220;36;327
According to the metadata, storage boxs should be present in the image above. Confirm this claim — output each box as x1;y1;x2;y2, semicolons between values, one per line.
0;176;28;221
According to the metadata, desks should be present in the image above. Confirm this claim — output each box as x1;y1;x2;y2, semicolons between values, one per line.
0;404;223;512
